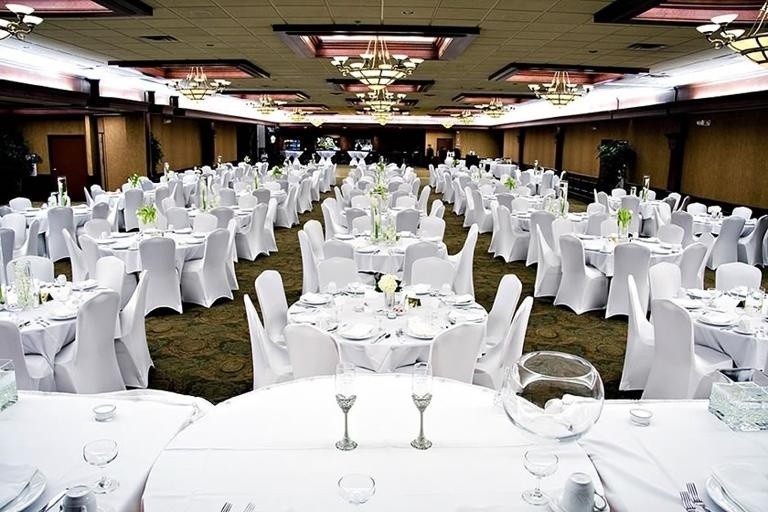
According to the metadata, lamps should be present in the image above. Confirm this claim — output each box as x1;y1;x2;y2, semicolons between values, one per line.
243;92;289;115
697;1;767;69
447;109;476;123
327;34;424;91
282;107;312;122
526;70;591;107
161;67;230;102
471;98;516;117
353;84;407;112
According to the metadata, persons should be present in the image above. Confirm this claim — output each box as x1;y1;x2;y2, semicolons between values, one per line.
426;144;447;160
260;150;269;162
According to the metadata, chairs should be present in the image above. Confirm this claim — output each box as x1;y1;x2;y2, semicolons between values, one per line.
0;148;767;512
